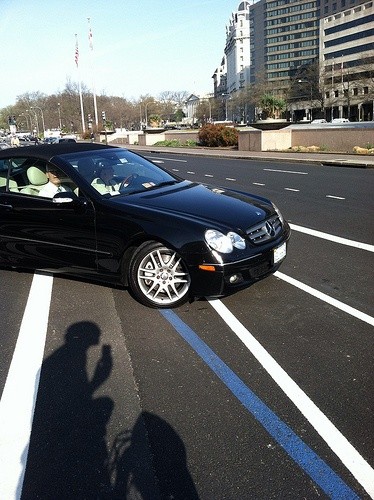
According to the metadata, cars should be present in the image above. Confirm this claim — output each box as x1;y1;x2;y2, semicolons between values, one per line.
0;142;291;310
0;133;38;149
52;138;77;144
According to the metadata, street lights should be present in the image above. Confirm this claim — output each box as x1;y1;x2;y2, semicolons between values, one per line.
145;102;156;125
298;80;313;122
18;106;45;132
222;93;235;122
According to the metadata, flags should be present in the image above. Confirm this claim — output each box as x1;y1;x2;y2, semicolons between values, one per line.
88;27;93;49
74;44;79;67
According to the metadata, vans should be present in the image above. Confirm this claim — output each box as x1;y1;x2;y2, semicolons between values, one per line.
16;130;31;137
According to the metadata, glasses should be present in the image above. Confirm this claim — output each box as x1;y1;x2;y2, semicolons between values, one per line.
47;168;60;173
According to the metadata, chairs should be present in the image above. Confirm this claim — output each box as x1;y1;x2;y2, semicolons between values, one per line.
20;164;49;196
78;158;94;184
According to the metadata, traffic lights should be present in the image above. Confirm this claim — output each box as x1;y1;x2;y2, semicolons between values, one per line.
101;111;106;120
7;116;12;122
13;115;16;120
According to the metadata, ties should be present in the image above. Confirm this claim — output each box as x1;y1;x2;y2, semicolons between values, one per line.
57;188;61;193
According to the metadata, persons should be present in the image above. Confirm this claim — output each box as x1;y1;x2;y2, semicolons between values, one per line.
11;134;19;148
34;135;39;145
90;160;130;195
39;161;73;198
